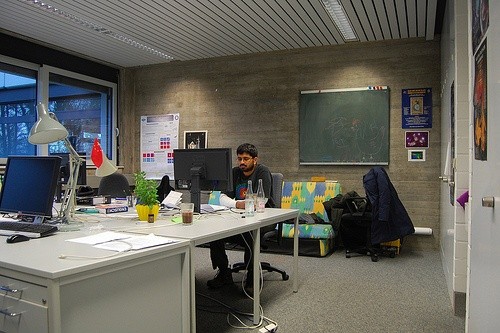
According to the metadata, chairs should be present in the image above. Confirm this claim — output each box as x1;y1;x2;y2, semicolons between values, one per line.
232;173;289;287
99;173;130;197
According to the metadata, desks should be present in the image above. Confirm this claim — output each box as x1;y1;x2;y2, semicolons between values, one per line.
0;201;301;333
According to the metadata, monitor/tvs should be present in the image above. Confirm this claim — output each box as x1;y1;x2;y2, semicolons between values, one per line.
173;147;233;215
50;152;86;201
0;156;62;224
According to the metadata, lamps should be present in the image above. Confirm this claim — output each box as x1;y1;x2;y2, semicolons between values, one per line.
27;103;85;225
69;139;118;223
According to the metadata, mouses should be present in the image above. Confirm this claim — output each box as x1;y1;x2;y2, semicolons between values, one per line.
6;234;30;243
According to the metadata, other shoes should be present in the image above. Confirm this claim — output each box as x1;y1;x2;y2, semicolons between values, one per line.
207;268;234;288
246;272;254;288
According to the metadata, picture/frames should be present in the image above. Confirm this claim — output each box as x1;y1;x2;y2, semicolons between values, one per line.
183;130;208;149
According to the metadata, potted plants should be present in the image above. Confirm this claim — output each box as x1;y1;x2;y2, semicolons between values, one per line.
133;170;160;220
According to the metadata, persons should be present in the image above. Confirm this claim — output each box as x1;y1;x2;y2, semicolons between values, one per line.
207;143;277;297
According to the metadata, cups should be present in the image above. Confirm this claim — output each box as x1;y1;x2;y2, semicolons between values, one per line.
181;203;194;225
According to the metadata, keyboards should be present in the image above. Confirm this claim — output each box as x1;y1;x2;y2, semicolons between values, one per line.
0;220;58;238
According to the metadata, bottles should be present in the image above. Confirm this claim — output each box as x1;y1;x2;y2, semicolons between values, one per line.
256;179;265;212
245;180;254;216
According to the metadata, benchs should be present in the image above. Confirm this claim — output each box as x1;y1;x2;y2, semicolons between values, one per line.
281;180;342;258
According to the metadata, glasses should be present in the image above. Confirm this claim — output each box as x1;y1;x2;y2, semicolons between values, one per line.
236;158;253;164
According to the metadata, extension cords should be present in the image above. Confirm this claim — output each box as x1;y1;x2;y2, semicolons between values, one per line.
258;323;276;333
